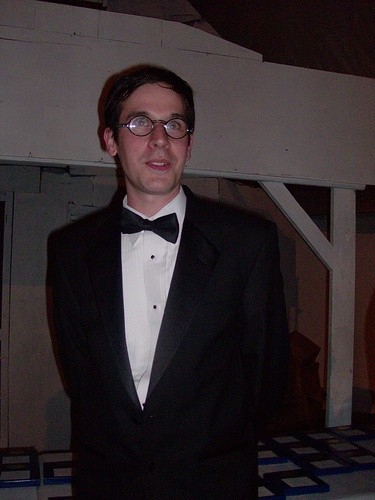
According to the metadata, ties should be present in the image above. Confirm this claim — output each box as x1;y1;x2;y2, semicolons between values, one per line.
119;207;179;244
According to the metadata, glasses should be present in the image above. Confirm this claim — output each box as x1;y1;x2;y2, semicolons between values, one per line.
115;114;194;139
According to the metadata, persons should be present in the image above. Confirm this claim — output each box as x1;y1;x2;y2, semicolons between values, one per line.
45;62;289;499
